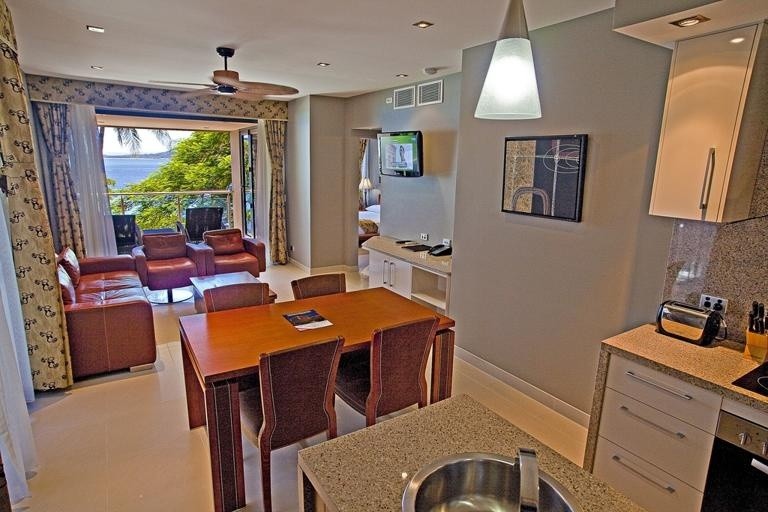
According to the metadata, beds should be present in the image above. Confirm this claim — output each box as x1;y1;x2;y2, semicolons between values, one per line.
358;210;383;248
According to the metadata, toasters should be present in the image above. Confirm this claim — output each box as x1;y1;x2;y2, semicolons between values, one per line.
656;298;721;346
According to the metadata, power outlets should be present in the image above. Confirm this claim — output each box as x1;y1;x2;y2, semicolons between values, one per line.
699;294;729;315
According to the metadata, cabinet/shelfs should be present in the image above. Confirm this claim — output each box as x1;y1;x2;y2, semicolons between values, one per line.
593;350;724;511
365;248;452;317
647;18;766;225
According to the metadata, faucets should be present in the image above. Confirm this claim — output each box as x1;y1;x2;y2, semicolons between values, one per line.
512;448;540;512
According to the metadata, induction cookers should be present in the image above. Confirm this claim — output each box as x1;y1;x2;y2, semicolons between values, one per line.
729;360;768;398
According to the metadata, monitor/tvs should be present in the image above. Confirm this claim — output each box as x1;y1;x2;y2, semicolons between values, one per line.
376;130;423;178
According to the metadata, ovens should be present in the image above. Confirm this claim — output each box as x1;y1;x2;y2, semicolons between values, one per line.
698;410;768;511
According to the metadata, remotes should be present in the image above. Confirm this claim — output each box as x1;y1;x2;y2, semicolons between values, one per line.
405;241;419;245
396;240;411;244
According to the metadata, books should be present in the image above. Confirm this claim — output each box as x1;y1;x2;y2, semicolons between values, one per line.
282;309;333;332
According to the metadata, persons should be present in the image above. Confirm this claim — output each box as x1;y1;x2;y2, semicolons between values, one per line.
399;147;406;169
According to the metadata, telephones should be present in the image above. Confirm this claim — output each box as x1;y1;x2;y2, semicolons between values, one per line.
428;244;452;256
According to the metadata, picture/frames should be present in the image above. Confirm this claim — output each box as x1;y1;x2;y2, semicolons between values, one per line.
500;133;589;223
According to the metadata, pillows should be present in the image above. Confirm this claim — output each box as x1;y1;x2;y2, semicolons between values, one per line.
141;234;188;261
55;262;77;305
56;246;82;288
204;231;246;256
364;204;380;214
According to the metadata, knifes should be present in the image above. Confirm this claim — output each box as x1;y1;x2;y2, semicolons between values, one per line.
746;300;768;334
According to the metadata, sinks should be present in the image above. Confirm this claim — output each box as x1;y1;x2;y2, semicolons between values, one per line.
401;452;583;512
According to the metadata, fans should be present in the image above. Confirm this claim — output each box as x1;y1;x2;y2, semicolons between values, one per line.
140;46;300;107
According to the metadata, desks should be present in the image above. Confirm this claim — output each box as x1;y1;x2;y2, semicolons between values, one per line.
297;392;649;511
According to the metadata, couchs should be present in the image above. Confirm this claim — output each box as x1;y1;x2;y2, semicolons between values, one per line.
52;246;157;384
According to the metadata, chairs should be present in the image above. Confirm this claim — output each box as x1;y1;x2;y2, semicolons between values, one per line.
130;232;207;305
289;271;346;303
174;206;227;246
201;281;270;315
235;331;347;512
327;313;442;430
198;226;267;280
110;214;144;256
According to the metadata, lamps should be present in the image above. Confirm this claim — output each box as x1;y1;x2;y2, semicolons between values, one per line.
357;177;374;209
470;1;544;121
